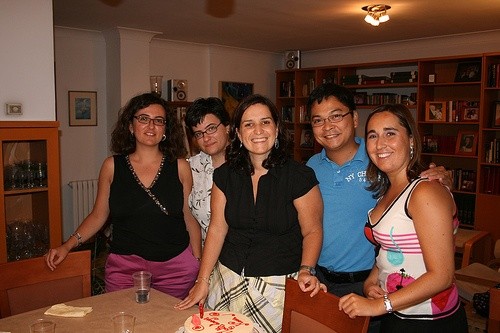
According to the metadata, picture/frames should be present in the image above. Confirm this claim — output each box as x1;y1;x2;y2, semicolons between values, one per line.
454;129;478;155
68;91;97;127
463;108;479;122
218;80;254;118
425;101;445;121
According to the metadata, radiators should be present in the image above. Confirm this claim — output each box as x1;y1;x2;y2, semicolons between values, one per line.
70;180;99;233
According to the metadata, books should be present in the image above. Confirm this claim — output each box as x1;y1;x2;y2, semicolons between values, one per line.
486;139;500;164
283;70;415;122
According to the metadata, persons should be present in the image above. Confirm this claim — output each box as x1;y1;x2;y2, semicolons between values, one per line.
304;83;454;298
183;98;236;260
175;95;327;333
43;93;200;296
301;131;312;145
338;104;469;333
429;103;479;152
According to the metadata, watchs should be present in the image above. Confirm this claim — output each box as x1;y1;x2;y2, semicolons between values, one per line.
301;265;316;276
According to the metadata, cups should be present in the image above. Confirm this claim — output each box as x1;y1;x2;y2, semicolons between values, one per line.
7;159;48;190
132;270;151;304
111;311;136;333
29;318;56;333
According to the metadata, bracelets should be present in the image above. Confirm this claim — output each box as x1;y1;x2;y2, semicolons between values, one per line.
196;279;210;285
384;295;394;314
70;232;83;248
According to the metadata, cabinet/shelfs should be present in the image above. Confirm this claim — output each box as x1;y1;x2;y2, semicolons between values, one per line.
0;121;62;262
275;53;500;228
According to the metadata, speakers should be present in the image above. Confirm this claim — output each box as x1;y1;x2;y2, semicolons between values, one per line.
168;79;189;101
286;50;301;69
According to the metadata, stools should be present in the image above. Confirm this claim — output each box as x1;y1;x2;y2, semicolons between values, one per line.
454;231;491;268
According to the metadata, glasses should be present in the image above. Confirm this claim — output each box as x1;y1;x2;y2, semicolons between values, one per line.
133;115;167;126
309;112;351;127
189;123;222;140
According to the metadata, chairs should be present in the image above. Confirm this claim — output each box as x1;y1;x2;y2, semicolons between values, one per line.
283;278;370;333
0;250;91;319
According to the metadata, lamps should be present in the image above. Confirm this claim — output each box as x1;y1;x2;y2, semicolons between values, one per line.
361;4;390;27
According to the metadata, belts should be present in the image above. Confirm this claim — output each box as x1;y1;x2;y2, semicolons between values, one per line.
317;265;372;284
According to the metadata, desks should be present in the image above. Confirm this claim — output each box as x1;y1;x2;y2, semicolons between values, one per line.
0;288;212;333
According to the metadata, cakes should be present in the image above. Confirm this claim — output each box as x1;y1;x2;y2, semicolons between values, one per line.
183;310;254;333
